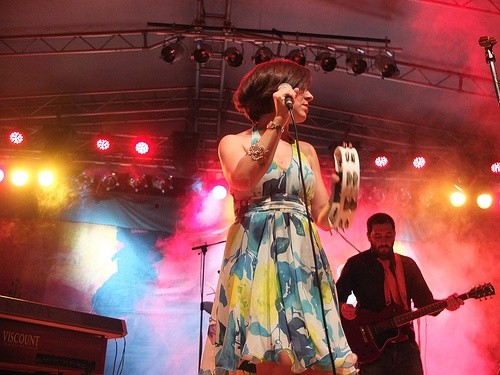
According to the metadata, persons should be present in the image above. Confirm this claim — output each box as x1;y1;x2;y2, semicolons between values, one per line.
336;212;465;375
198;58;357;375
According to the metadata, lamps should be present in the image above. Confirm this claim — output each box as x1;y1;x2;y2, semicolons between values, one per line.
191;38;212;65
373;50;400;79
252;40;274;67
286;45;308;66
314;46;338;71
161;33;189;64
344;45;368;75
222;37;245;70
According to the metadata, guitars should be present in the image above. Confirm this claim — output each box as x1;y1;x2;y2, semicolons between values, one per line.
343;281;495;362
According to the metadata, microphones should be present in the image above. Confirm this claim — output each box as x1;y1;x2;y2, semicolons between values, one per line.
285;96;293;110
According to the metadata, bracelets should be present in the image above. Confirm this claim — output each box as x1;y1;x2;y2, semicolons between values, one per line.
266;120;285;133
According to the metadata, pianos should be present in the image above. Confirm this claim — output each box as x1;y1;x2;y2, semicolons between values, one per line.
0;295;128;375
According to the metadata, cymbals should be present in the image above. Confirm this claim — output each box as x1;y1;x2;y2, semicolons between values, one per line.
200;300;215;312
